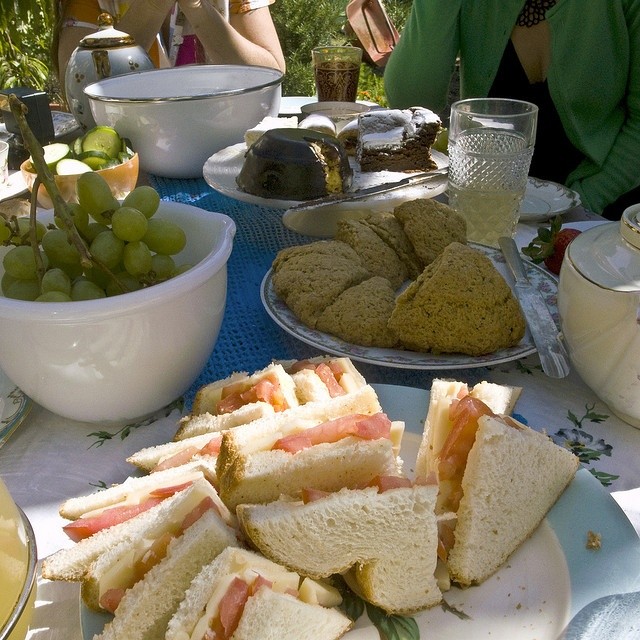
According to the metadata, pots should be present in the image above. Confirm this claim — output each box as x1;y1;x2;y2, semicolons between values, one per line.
557;202;639;430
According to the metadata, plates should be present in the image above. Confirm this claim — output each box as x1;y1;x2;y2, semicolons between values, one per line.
79;382;640;640
276;95;318;114
444;175;583;223
0;169;29;203
0;369;33;450
0;110;80;138
259;239;563;372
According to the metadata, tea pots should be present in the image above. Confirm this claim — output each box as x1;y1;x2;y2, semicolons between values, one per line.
64;12;155;135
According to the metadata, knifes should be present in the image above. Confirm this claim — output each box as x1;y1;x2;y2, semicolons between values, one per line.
289;166;449;212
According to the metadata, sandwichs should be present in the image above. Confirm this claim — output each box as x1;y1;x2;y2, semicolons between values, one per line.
0;352;581;640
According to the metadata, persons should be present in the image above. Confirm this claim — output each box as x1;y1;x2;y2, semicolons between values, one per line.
382;0;640;222
49;0;286;112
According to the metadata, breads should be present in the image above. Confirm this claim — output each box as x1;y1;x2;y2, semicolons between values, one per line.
335;115;362;157
235;126;354;202
356;105;442;172
298;111;338;138
242;114;298;149
267;196;528;358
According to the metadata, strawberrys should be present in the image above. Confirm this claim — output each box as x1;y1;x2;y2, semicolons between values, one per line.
520;213;583;277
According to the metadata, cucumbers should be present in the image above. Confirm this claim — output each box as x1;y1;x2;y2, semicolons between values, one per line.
27;124;135;177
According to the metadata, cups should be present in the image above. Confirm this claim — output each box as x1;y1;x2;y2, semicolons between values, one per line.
311;46;363;103
447;97;540;251
0;141;10;191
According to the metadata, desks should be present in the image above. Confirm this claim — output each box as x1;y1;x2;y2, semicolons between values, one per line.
276;89;378;113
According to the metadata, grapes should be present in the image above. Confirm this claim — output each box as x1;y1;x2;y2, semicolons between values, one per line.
0;92;195;303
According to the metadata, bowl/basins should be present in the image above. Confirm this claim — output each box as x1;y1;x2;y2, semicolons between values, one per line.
0;196;239;426
81;63;286;180
300;101;372;124
20;151;140;211
